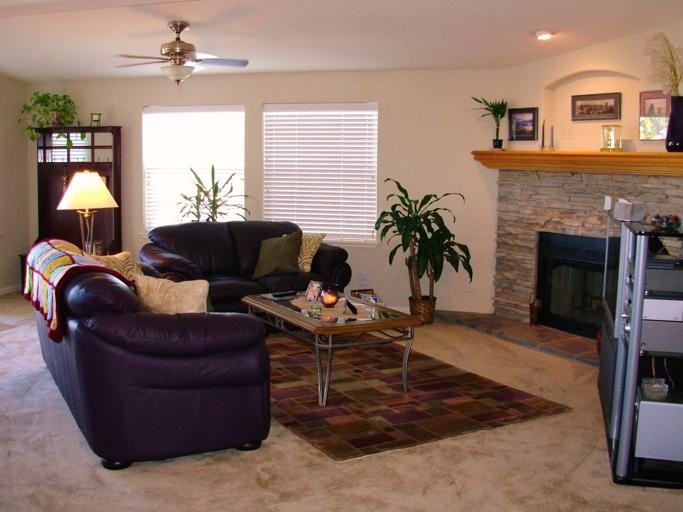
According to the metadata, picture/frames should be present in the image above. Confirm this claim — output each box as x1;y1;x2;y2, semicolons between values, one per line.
506;105;538;142
635;89;672;143
569;91;621;121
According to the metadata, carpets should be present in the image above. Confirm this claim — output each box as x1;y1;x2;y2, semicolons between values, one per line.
254;322;573;464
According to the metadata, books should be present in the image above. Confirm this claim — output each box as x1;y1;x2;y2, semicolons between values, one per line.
351;289;378;321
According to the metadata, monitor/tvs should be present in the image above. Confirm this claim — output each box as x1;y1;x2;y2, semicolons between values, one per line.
602;212;627;340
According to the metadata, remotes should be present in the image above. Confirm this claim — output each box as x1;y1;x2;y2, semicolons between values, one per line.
273;290;297;297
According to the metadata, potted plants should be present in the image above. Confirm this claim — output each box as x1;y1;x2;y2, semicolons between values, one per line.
467;93;508;149
17;88;84;146
369;176;472;324
645;32;682;151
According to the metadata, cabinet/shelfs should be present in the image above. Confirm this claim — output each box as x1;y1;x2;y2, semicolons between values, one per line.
32;122;123;252
590;207;682;490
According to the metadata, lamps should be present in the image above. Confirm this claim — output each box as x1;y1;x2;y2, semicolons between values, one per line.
317;282;339;309
88;111;101;126
152;61;200;88
53;171;118;255
532;27;552;42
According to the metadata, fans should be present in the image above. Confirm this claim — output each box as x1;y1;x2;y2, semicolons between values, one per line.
116;19;247;81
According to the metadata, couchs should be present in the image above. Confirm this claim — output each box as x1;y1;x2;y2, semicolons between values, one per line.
26;240;269;470
141;220;351;322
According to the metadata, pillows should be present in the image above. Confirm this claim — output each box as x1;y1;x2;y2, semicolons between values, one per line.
131;274;209;315
250;230;301;279
282;232;326;271
90;251;143;285
